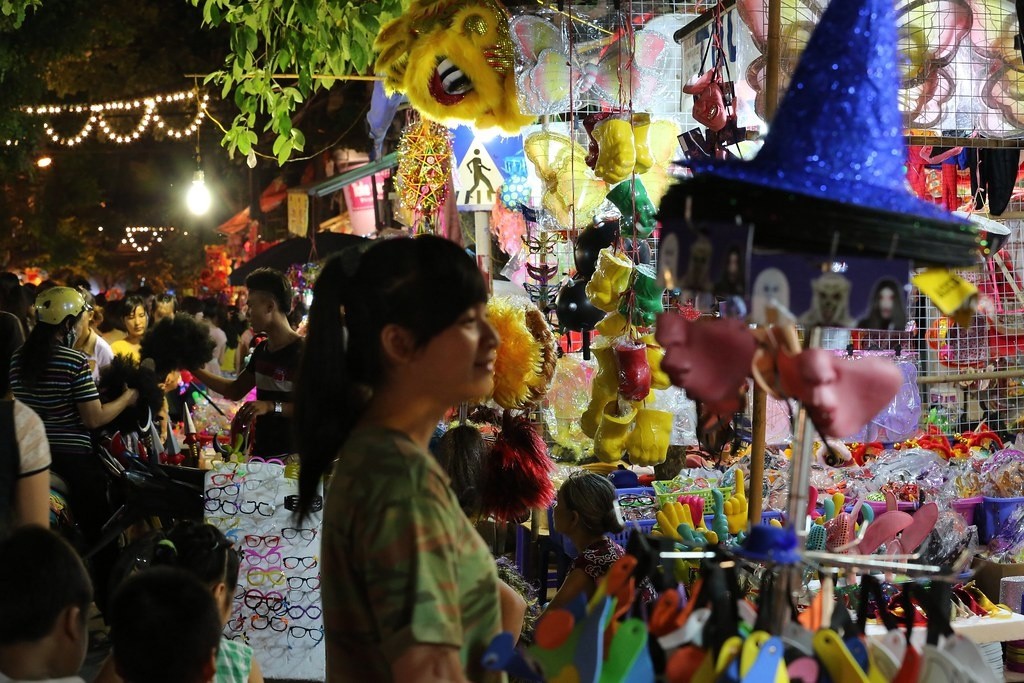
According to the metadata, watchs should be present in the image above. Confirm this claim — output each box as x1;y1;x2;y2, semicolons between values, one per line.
274;400;283;416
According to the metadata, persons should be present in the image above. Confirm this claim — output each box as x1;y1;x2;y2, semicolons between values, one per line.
752;268;907;331
293;235;526;683
656;234;741;297
0;269;302;683
532;473;667;675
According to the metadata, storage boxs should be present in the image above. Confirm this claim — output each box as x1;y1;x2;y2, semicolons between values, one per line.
553;485;1024;560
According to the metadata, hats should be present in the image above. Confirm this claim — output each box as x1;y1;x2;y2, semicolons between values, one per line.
653;1;1013;264
733;523;801;563
228;231;365;287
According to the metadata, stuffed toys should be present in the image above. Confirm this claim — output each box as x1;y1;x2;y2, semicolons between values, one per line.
372;0;538;135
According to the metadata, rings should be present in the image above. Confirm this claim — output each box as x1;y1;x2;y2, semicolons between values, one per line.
242;406;246;410
248;412;253;415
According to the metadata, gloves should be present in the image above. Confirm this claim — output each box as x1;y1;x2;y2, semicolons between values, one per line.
557;114;674;467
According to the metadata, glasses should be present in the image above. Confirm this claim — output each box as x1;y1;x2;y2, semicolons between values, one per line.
201;455;326;650
569;469;596;511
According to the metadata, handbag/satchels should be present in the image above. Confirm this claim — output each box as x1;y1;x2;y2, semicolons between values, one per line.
682;68;726;129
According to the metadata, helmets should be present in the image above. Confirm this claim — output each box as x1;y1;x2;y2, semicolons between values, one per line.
34;287;88;324
